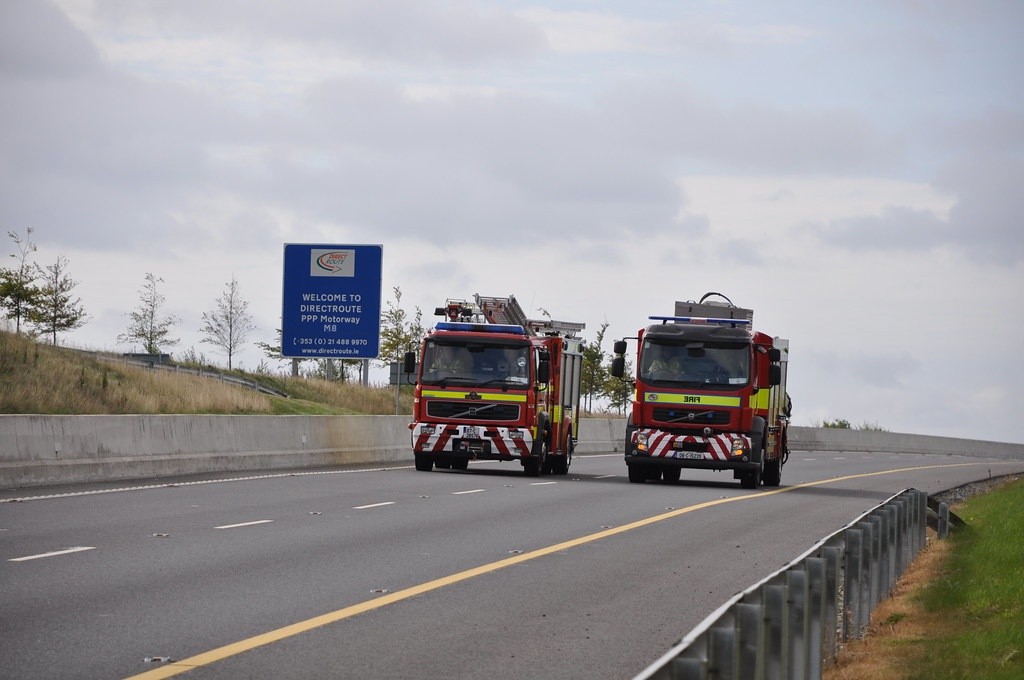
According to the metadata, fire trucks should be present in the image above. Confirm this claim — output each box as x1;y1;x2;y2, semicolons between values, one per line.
609;292;792;490
407;290;588;478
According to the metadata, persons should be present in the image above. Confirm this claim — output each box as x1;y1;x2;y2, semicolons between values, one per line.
493;348;524;379
710;351;744;383
432;346;463;373
648;348;683;378
